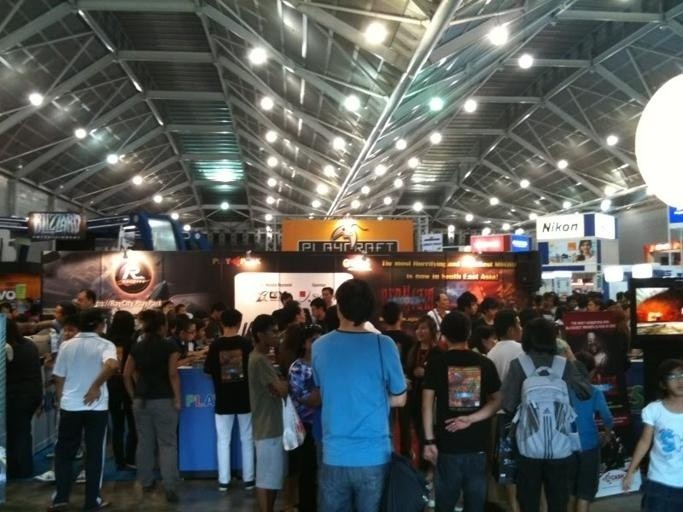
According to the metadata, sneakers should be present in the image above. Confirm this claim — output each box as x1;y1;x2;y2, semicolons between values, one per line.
45;502;70;512
82;501;112;512
74;447;85;461
141;481;156;492
217;482;231;492
32;470;55;483
242;481;256;491
166;490;178;503
75;468;87;485
113;461;137;474
44;449;55;459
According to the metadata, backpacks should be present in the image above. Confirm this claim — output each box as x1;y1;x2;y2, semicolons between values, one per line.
512;351;581;460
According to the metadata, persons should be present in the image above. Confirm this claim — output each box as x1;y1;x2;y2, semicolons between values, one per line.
2;288;225;510
577;241;593;260
203;280;683;510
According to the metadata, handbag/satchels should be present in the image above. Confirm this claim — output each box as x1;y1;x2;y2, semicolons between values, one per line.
598;425;629;472
381;449;430;512
